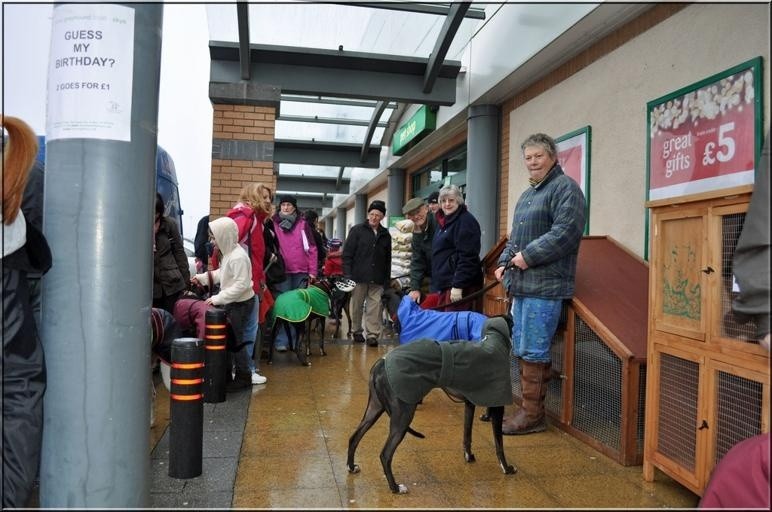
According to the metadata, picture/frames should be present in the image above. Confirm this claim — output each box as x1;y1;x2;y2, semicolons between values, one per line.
553;122;592;236
643;55;765;261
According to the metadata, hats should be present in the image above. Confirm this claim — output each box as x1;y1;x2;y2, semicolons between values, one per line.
280;196;297;207
368;200;386;215
402;197;424;215
428;192;440;203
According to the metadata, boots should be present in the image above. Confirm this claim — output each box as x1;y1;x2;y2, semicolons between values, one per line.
502;358;551;435
226;369;252;392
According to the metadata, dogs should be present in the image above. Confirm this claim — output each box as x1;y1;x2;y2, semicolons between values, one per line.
168;284;253;384
381;279;491;422
266;273;348;365
346;313;517;494
312;274;351;338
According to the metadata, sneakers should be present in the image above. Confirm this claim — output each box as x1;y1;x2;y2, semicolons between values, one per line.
251;373;266;384
367;337;378;347
353;334;365;342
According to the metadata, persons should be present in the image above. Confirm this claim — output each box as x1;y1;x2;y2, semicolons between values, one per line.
304;210;343;319
193;183;286;391
19;156;42;339
342;200;392;347
730;131;772;355
699;431;772;510
403;184;482;311
155;192;191;314
494;131;588;437
0;114;53;507
269;194;317;351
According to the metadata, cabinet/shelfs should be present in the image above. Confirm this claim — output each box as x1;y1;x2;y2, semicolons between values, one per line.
637;184;772;498
